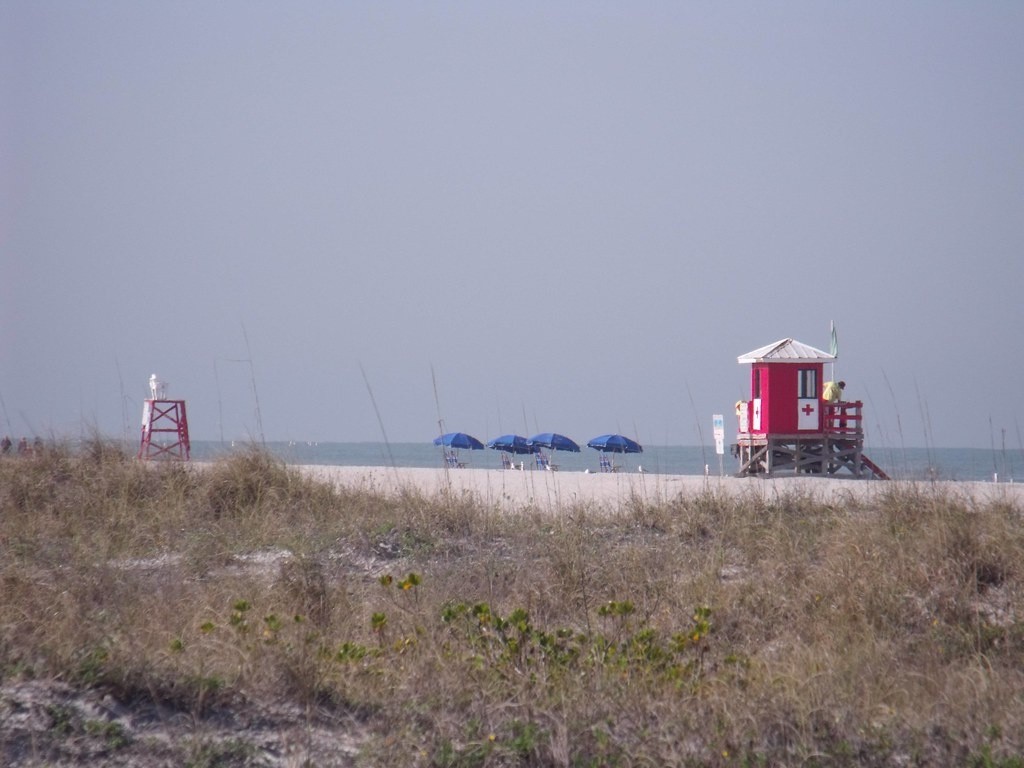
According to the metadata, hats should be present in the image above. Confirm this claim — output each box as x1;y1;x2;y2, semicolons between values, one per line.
839;381;846;390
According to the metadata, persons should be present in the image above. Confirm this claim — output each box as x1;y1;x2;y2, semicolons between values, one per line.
18;437;28;453
1;435;12;453
33;436;44;456
822;381;846;428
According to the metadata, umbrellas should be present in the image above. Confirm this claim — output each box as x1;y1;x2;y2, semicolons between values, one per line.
434;432;643;471
